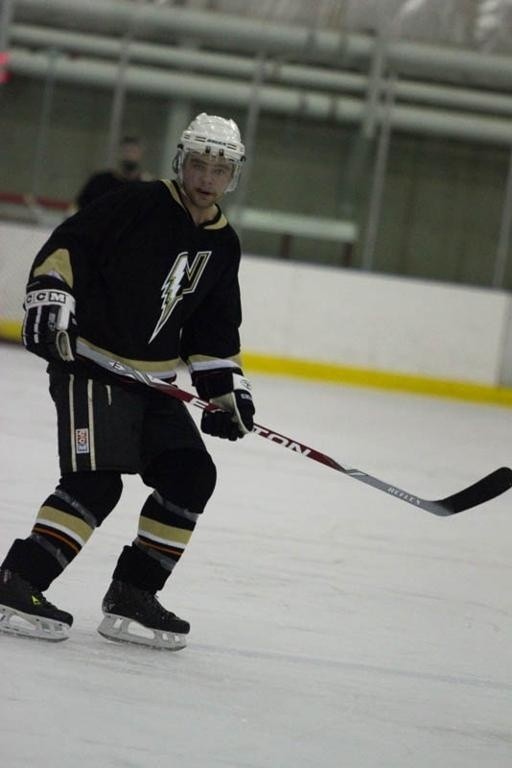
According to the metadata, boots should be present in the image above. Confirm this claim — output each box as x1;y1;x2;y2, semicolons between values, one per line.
102;543;191;633
0;538;75;626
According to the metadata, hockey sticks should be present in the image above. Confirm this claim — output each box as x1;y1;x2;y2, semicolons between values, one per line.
72;337;511;516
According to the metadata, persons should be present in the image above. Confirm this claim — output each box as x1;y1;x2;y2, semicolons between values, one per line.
0;113;257;633
65;137;155;219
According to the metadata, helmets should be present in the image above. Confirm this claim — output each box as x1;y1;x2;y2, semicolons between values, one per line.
172;112;246;194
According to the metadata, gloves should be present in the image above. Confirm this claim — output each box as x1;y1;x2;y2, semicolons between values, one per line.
188;366;254;441
21;277;81;369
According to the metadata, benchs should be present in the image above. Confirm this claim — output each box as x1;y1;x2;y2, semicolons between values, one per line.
229;206;359;267
